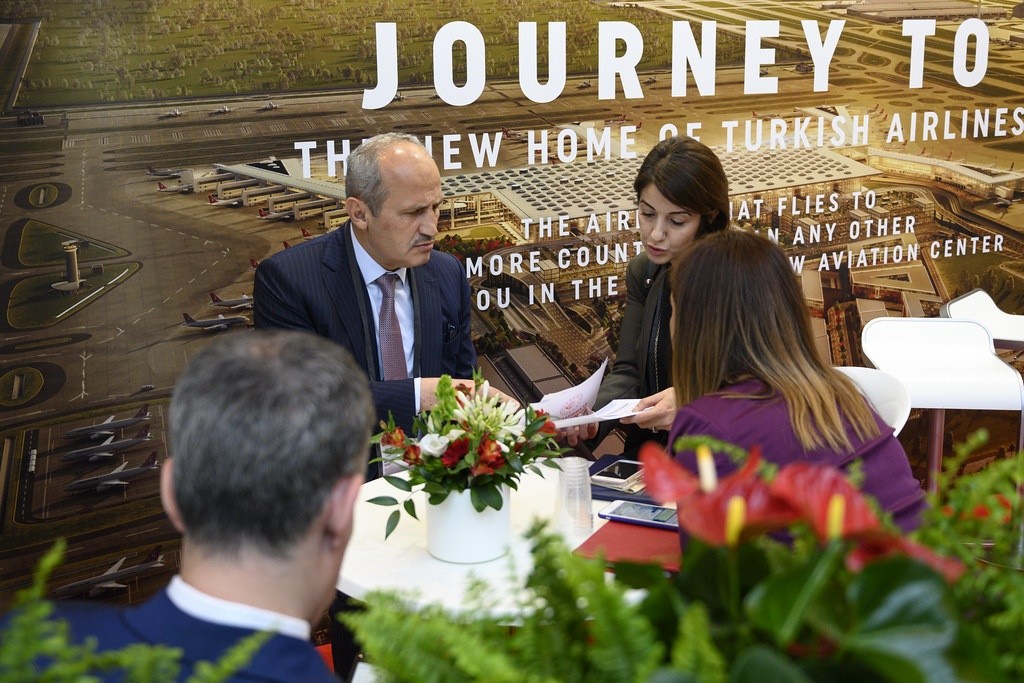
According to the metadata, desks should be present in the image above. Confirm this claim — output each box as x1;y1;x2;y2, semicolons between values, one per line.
334;457;670;629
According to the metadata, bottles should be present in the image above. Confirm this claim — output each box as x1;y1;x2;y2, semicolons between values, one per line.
558;456;594;539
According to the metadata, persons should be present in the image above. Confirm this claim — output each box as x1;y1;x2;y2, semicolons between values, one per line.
553;135;731;462
1;329;376;682
665;230;931;538
251;131;521;483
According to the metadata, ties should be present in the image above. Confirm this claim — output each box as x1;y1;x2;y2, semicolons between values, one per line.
369;273;409;381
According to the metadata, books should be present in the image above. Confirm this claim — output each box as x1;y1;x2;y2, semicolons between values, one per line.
572;519;682;580
588;454;664;506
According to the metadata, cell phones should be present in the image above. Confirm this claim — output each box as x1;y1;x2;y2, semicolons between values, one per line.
598;498;680;529
588;458;646;485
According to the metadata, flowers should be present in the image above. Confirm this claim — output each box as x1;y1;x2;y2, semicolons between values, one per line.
367;365;572;541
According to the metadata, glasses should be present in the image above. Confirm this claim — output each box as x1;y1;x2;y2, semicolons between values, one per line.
444;324;457;342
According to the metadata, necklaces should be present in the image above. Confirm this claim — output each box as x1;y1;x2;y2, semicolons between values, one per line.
654;321;661;392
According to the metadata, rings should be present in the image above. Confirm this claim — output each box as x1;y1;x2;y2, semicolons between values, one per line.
652;426;658;433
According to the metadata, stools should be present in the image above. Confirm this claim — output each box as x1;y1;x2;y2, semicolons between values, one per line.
861;316;1024;502
830;363;912;437
939;288;1024;351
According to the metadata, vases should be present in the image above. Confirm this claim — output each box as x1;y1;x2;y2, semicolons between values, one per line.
423;482;514;563
